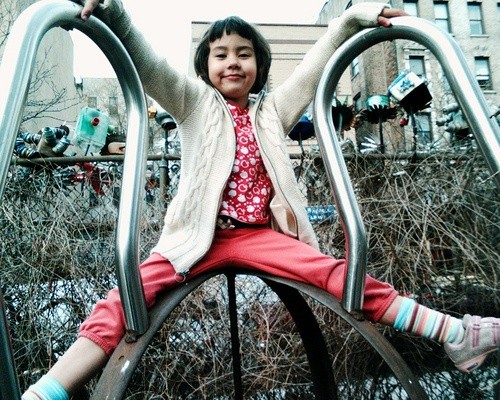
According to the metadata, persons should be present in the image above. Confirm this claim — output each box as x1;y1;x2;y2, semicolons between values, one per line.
20;0;500;400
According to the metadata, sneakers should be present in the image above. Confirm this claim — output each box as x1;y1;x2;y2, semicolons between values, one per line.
445;314;500;372
